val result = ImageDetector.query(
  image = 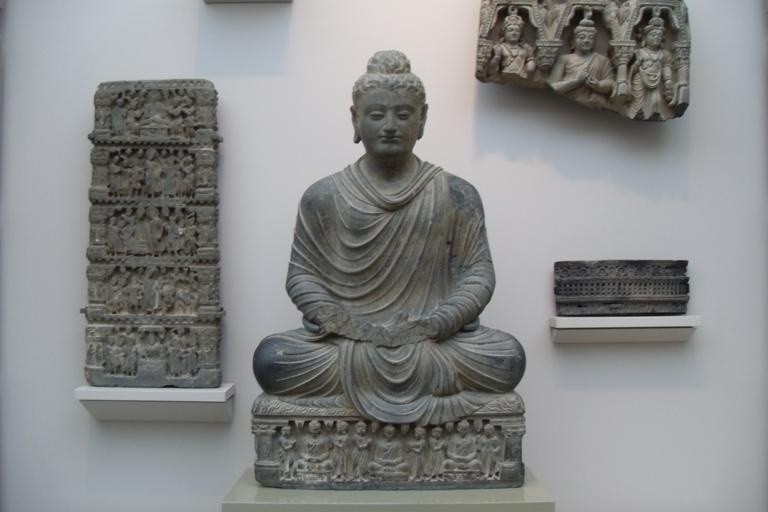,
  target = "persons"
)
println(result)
[104,91,199,378]
[252,50,526,396]
[489,6,536,78]
[626,16,676,119]
[547,7,615,109]
[274,419,503,485]
